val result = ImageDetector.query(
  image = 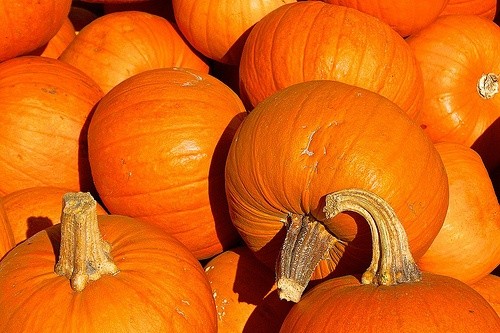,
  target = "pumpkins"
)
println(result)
[0,0,500,333]
